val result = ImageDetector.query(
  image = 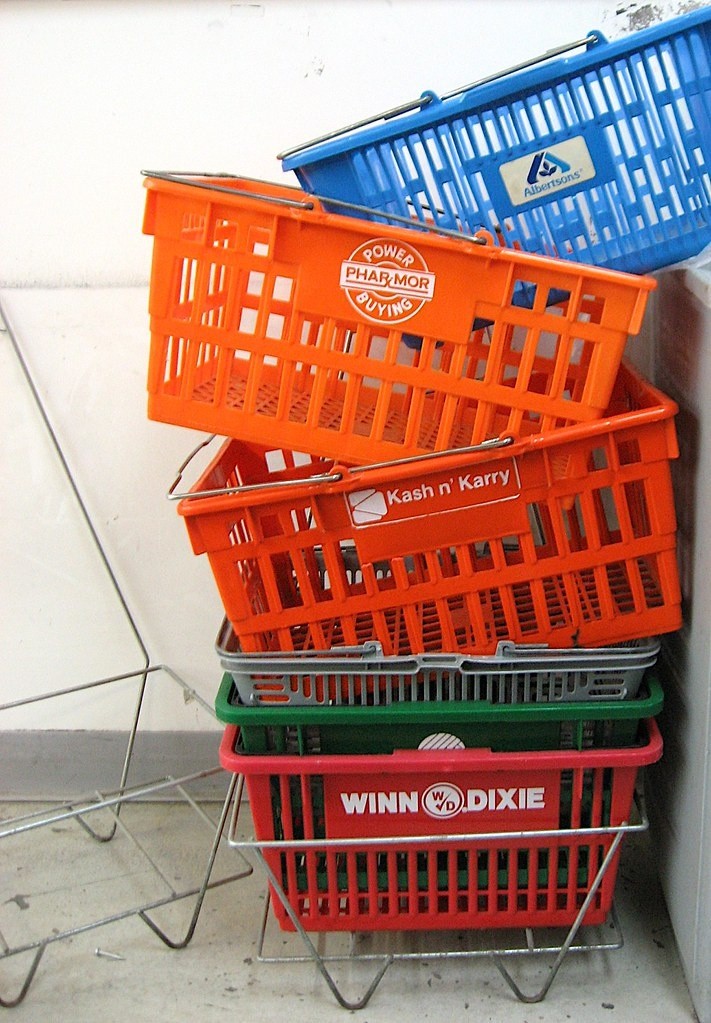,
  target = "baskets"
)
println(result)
[140,2,711,935]
